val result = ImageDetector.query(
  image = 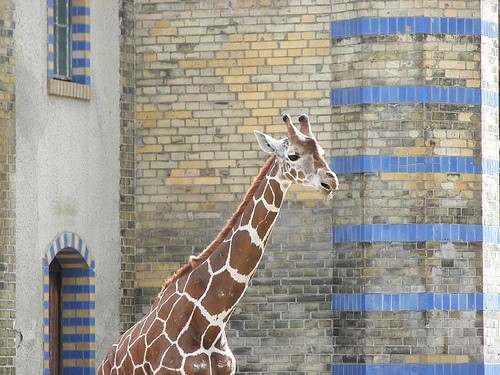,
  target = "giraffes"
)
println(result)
[97,114,339,375]
